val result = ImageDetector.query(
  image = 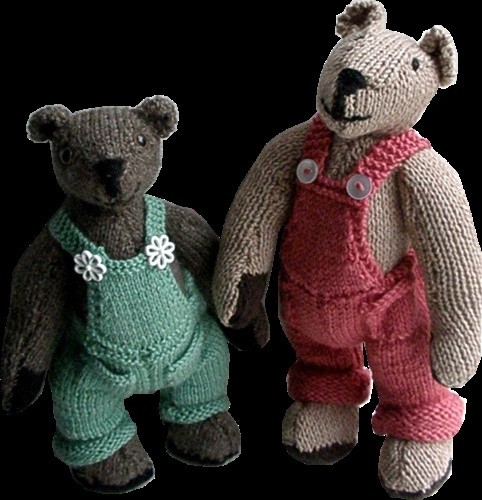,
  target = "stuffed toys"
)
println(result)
[212,0,481,500]
[0,95,241,494]
